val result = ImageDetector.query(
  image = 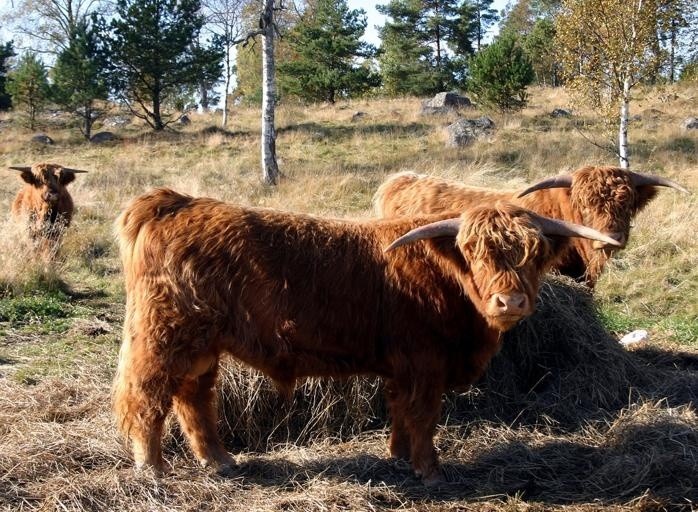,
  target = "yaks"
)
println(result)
[104,187,626,486]
[367,166,693,290]
[11,162,89,275]
